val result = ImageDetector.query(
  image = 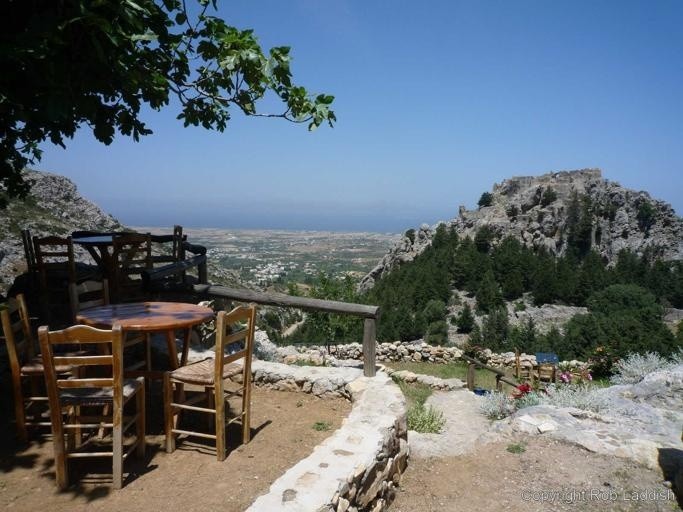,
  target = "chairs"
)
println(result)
[1,293,87,447]
[164,305,256,463]
[515,346,533,381]
[534,364,555,383]
[20,223,211,384]
[37,323,146,490]
